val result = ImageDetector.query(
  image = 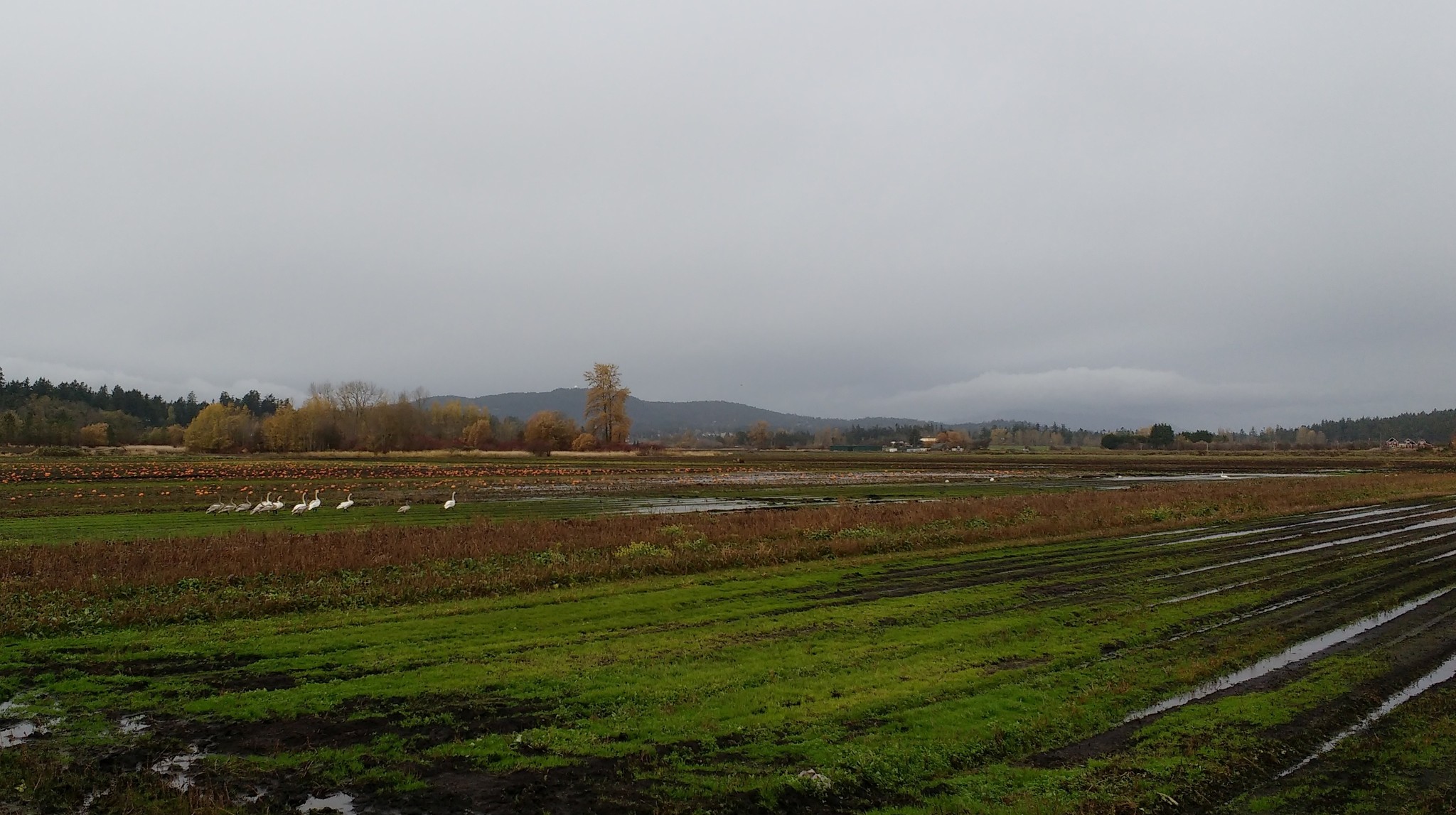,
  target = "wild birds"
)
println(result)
[945,479,950,483]
[1220,470,1230,480]
[217,496,237,516]
[307,489,321,514]
[396,498,412,515]
[443,491,457,511]
[235,493,252,516]
[249,491,284,516]
[290,491,310,517]
[205,494,225,517]
[990,478,995,482]
[335,492,354,513]
